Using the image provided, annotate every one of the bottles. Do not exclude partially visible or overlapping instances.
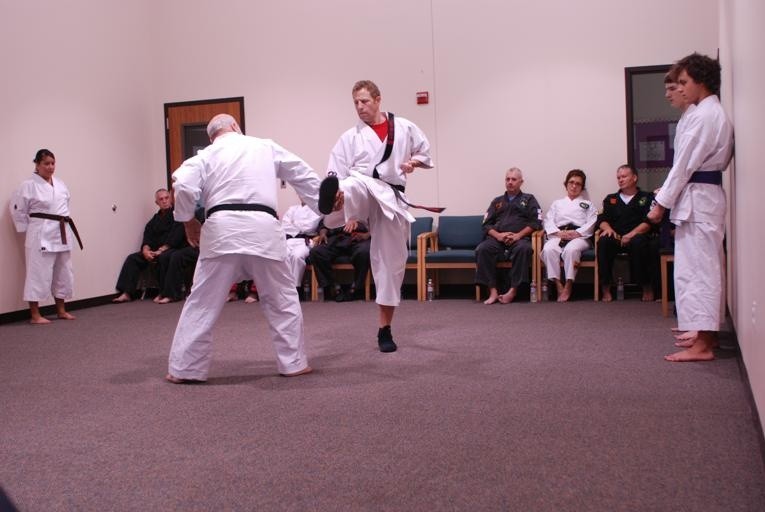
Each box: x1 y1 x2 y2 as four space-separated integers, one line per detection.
318 284 324 302
304 280 311 301
425 277 434 301
541 277 549 302
530 280 538 305
616 277 625 300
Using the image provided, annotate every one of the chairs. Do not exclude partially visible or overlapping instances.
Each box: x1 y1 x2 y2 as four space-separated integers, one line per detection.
312 255 366 303
478 215 537 304
365 217 433 302
537 226 602 303
610 252 644 296
660 246 675 307
422 213 486 302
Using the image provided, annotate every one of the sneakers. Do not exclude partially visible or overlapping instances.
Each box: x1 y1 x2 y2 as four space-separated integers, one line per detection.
378 325 397 352
318 171 338 214
348 280 359 301
331 284 345 302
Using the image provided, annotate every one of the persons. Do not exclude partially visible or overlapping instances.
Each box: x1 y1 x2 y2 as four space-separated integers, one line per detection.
474 168 543 305
282 192 322 300
226 279 260 304
9 147 83 325
157 206 206 304
112 187 174 304
166 113 343 385
597 163 658 304
537 168 600 302
309 198 373 303
664 53 737 364
315 79 433 354
662 70 699 341
